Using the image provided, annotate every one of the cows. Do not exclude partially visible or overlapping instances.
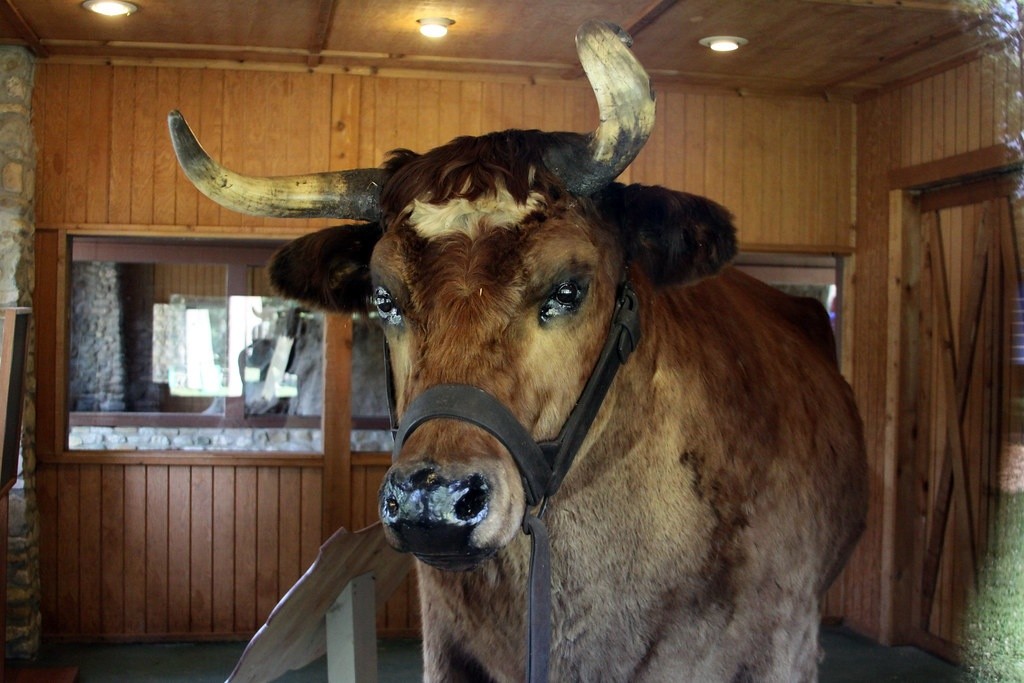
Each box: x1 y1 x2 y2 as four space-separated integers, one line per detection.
165 17 873 683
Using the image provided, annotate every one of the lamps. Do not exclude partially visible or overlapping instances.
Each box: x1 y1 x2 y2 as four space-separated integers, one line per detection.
698 36 749 52
415 16 456 38
